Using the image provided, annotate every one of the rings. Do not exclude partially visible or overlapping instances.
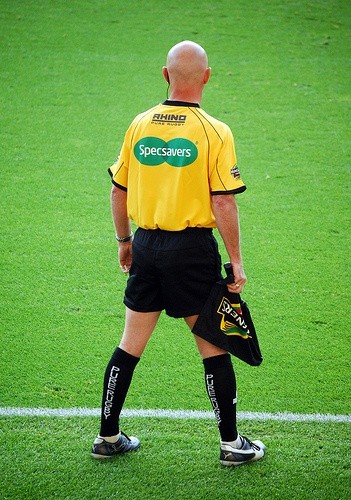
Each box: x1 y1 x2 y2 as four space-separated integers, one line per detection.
236 285 239 288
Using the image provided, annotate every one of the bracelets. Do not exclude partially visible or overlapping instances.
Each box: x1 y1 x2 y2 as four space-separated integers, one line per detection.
115 231 134 243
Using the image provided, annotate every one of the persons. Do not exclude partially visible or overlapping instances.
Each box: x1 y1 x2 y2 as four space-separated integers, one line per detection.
90 40 266 467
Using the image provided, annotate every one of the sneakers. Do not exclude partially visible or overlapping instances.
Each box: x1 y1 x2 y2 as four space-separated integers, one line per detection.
90 431 139 458
220 436 265 466
